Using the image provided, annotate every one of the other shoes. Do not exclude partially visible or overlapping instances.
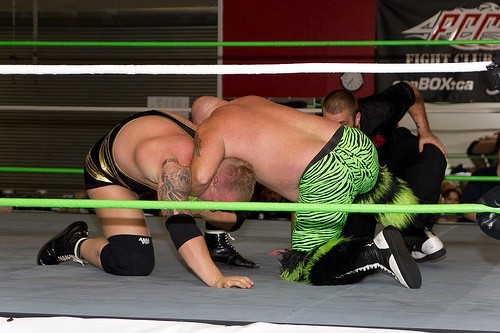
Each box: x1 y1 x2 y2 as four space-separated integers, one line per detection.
38 219 90 266
404 226 447 261
374 225 421 289
203 231 260 268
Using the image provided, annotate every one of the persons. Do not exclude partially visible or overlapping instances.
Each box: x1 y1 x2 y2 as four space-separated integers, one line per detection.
269 80 447 261
37 110 255 289
439 131 500 222
190 96 422 290
0 188 93 214
